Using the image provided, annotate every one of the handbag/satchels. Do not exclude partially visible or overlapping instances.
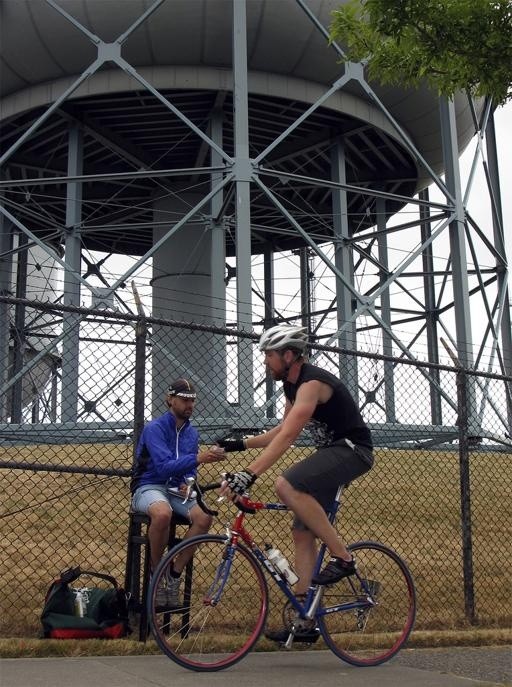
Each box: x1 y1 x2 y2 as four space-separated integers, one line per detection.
40 567 127 639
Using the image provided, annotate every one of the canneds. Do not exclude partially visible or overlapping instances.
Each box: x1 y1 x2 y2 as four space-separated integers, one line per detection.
74 597 87 618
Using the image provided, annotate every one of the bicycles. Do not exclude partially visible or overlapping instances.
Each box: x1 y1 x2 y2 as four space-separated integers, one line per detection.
145 478 418 673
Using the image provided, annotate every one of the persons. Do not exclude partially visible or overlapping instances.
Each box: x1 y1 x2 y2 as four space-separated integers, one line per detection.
129 378 225 607
213 324 375 643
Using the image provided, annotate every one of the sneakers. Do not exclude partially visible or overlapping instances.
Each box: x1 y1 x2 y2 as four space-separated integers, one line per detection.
166 564 183 609
310 555 357 585
150 567 167 612
264 624 320 644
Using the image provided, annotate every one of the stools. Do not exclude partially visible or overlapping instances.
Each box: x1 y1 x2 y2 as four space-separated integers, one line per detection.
126 512 194 642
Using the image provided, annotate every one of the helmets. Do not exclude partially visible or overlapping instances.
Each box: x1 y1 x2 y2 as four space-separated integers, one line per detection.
257 324 316 349
166 380 197 398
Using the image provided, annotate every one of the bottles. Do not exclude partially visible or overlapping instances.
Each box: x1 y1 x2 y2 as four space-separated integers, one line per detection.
263 543 301 588
